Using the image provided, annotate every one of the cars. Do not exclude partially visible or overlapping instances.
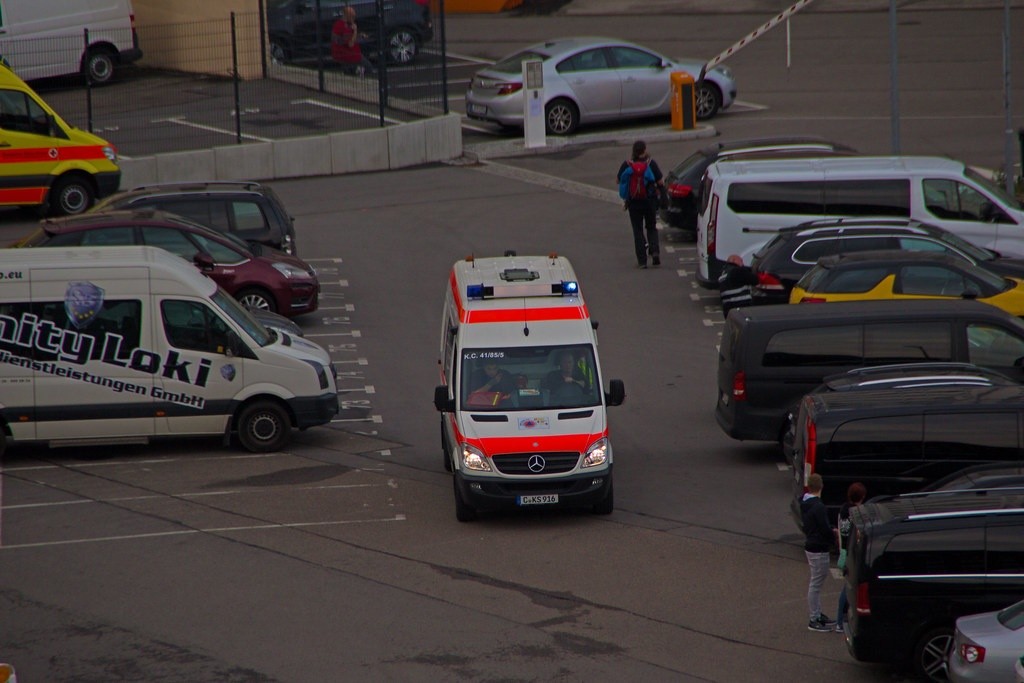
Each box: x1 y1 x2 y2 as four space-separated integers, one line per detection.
78 288 302 353
465 35 736 136
780 362 1024 683
659 136 886 228
790 250 1024 353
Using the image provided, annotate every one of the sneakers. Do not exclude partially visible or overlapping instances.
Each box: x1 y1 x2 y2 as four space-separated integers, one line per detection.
808 618 833 632
821 613 838 625
835 623 843 632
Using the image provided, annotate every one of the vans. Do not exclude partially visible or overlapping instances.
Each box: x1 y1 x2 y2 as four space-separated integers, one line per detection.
0 245 339 467
696 156 1024 291
714 301 1024 465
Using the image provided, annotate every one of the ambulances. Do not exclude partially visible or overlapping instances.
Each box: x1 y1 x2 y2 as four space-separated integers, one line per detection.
433 249 625 525
0 63 122 214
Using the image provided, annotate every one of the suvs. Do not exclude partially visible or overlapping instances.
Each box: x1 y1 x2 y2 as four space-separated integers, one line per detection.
752 216 1024 305
16 208 319 318
85 180 297 257
262 0 432 66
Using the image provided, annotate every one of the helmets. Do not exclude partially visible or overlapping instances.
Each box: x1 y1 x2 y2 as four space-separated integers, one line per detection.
343 7 355 16
727 255 743 267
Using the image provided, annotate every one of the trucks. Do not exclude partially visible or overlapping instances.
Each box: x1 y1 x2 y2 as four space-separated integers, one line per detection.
0 0 145 87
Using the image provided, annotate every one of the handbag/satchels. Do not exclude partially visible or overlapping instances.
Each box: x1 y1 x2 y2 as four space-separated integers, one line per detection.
465 391 503 407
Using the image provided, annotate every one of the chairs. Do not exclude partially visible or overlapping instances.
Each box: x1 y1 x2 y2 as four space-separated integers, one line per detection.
546 351 590 395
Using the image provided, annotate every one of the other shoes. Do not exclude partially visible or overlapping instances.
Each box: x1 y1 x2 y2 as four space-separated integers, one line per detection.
640 264 647 269
652 253 661 266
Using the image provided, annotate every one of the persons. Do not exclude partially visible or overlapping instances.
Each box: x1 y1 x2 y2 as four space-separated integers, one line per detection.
541 351 591 396
472 356 516 399
800 473 867 632
331 6 374 77
616 141 665 268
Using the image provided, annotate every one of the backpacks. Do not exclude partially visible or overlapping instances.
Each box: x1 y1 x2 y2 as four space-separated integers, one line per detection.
619 158 655 211
